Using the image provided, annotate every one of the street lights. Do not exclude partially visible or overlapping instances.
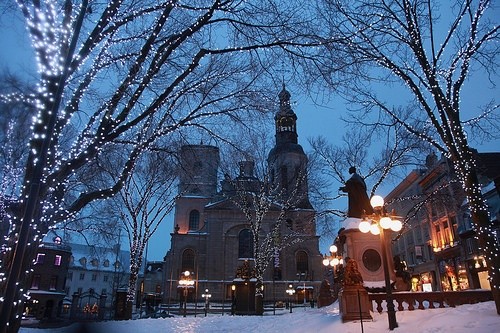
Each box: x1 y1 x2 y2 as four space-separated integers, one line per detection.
321 245 345 300
359 194 403 332
201 289 212 317
286 285 296 313
178 270 194 317
